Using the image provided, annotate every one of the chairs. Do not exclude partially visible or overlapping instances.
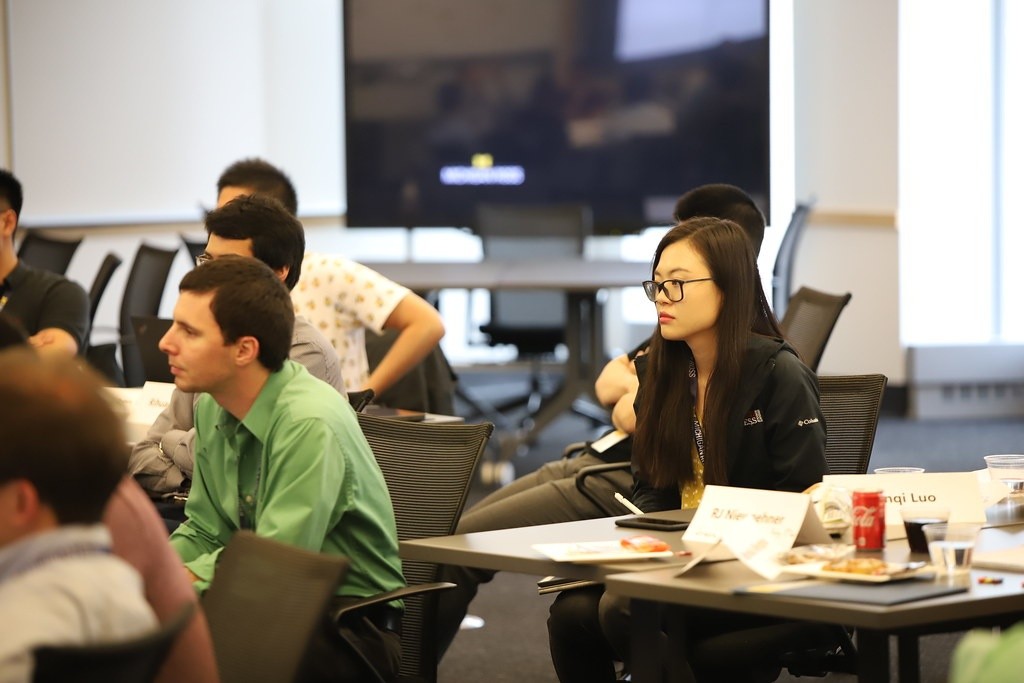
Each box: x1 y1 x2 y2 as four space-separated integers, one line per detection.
13 229 212 390
561 286 851 519
197 535 350 682
474 199 607 443
622 371 890 683
30 594 205 682
307 417 500 683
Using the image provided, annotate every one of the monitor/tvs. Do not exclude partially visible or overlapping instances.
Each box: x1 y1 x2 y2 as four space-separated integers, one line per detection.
342 0 771 230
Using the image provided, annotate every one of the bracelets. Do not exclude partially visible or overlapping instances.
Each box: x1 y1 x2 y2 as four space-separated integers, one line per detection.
159 440 164 456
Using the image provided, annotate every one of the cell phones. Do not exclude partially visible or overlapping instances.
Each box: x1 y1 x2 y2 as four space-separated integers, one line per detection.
616 517 691 531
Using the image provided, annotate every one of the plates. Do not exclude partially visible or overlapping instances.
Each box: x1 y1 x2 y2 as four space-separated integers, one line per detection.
779 558 927 582
534 538 673 565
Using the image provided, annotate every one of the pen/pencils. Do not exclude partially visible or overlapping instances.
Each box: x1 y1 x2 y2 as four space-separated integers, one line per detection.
615 492 644 514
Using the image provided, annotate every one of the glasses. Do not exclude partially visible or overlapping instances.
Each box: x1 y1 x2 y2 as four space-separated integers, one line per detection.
196 255 208 266
643 278 711 304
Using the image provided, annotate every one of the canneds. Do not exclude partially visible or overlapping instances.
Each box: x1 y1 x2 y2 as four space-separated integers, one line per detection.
852 488 886 551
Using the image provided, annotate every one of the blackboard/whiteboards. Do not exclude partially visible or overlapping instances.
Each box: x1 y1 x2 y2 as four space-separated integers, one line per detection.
0 0 352 236
793 0 897 228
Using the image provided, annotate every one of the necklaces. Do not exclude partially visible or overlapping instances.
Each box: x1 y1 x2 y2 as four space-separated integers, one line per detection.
238 442 261 529
689 353 706 467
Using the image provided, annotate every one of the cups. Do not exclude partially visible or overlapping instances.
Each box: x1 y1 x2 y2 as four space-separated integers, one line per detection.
874 466 950 552
923 522 980 585
984 453 1024 506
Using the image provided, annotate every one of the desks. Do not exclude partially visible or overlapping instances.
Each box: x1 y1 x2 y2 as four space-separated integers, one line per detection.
399 471 1024 683
372 257 657 459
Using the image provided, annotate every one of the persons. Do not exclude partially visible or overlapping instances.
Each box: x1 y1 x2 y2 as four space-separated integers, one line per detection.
0 169 218 683
424 184 765 665
129 158 445 536
159 256 407 683
547 217 827 683
